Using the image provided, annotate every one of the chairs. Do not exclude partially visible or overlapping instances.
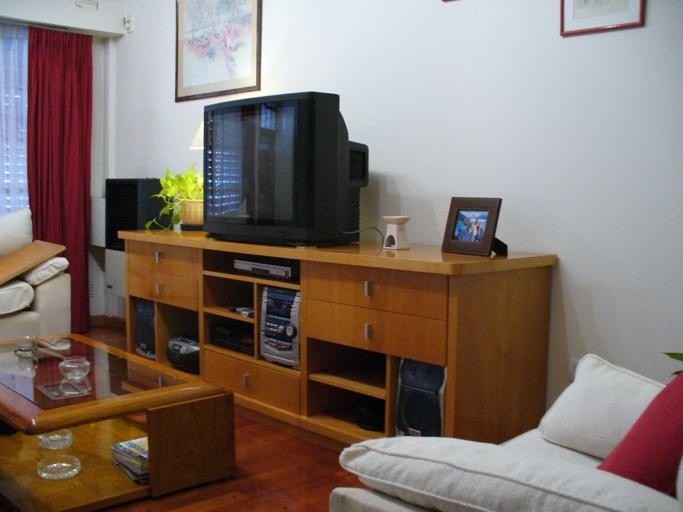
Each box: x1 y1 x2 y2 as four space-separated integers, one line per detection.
329 353 683 511
0 206 72 345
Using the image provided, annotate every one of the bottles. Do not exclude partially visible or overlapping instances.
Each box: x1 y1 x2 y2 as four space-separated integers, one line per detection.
59 379 94 398
58 356 92 381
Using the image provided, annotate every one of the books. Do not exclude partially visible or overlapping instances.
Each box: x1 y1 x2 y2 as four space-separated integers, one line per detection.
111 435 149 484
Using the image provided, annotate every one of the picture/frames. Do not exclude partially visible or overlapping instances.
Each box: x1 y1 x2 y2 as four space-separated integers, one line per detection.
559 0 646 37
440 197 508 258
175 0 262 103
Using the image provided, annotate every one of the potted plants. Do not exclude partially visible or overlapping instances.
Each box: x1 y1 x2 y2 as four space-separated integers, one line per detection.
145 162 204 229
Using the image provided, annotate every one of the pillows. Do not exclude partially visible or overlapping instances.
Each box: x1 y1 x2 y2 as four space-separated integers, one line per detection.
595 370 683 499
538 352 667 462
23 257 70 285
0 280 35 316
339 435 683 511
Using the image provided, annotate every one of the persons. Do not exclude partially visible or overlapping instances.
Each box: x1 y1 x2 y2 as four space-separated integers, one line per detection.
455 217 465 240
469 223 482 242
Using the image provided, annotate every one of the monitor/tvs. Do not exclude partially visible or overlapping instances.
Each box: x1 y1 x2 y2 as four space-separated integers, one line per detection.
202 90 369 249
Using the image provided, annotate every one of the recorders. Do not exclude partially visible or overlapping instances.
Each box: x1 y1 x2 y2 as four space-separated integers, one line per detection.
167 335 200 374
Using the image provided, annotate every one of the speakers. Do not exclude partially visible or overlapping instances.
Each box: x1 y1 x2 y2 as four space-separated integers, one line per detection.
394 358 446 437
134 298 156 360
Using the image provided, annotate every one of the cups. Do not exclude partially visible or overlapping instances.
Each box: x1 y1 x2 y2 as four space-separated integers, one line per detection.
17 334 39 352
17 354 40 373
38 429 75 468
